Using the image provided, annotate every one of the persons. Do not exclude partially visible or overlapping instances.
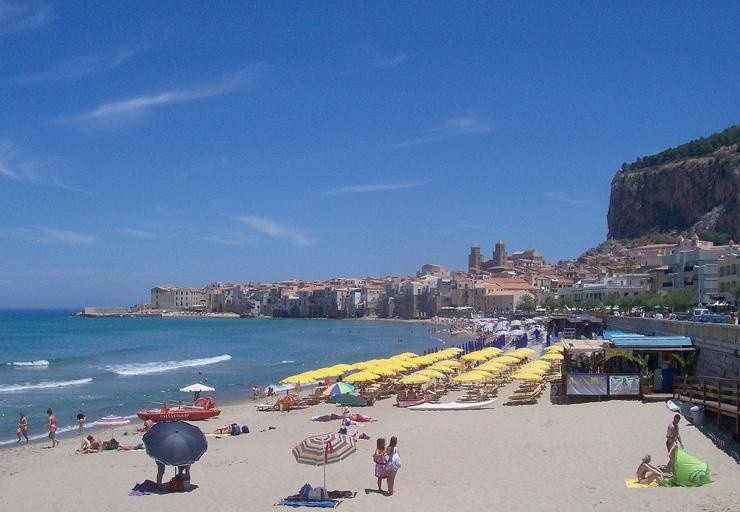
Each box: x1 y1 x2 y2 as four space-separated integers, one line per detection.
252 386 275 400
204 395 216 410
636 414 685 486
337 405 372 440
374 438 389 492
178 466 190 475
203 378 208 386
76 403 169 454
388 437 401 495
728 312 738 325
16 412 28 443
46 407 60 448
698 300 702 309
155 460 165 485
286 397 292 412
213 423 240 436
616 377 638 394
609 309 678 321
195 392 200 400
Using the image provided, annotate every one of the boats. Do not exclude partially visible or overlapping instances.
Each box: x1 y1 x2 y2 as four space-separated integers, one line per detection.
134 396 222 423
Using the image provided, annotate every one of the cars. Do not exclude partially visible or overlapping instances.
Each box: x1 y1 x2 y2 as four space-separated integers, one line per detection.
535 302 733 323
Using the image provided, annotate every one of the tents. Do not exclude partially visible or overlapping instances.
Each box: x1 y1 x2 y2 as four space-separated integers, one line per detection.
664 445 711 488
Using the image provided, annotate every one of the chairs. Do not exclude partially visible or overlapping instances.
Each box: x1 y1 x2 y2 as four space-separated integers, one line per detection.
254 369 548 413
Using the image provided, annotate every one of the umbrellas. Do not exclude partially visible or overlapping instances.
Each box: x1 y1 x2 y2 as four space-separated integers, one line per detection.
291 432 356 488
279 316 569 396
141 421 208 467
179 383 215 397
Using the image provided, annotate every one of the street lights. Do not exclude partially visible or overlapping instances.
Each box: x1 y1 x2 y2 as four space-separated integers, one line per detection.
692 264 706 308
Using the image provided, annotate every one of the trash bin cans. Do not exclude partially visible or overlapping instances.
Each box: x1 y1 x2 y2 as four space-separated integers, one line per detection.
691 405 706 426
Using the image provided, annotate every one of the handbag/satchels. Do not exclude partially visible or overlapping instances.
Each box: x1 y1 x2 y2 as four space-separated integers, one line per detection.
170 480 183 491
231 423 240 435
242 425 249 433
392 453 401 469
103 439 119 450
299 484 329 500
